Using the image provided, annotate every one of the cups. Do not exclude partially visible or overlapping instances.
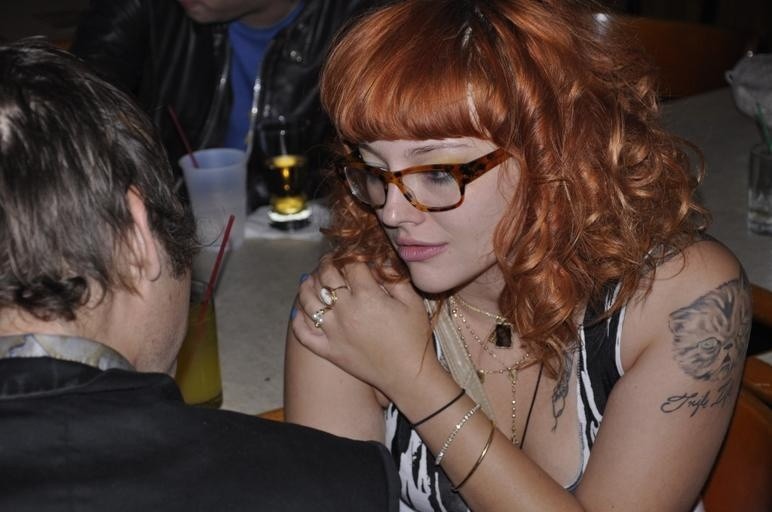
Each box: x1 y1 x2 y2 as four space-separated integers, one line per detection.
178 146 252 253
263 143 310 216
743 141 772 236
173 276 225 411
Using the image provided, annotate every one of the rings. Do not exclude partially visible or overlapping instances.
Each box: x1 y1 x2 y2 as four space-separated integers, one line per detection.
313 306 332 327
318 285 348 306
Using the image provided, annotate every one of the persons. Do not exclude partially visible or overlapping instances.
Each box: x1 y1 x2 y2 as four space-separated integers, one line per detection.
73 0 396 214
1 34 402 512
282 0 755 510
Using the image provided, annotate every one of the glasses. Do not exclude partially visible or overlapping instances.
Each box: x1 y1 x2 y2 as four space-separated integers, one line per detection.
337 146 511 212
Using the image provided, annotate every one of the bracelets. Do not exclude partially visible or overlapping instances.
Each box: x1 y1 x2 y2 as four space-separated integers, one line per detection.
435 404 482 466
409 389 466 430
451 421 496 492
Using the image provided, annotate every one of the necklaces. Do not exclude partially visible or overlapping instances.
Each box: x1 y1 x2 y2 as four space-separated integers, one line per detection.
450 295 549 451
